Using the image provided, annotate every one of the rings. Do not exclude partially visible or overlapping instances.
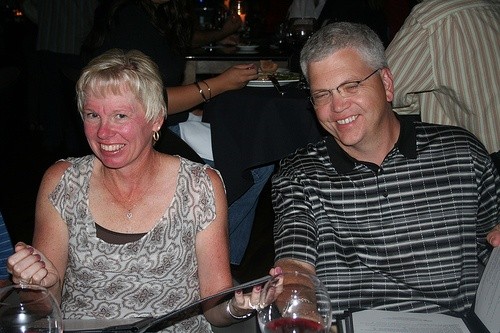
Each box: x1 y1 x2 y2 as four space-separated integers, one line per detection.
18 279 29 290
249 300 260 310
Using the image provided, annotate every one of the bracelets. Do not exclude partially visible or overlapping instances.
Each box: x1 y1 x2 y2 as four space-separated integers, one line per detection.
281 297 317 317
208 32 213 43
220 28 228 37
193 81 210 102
202 80 212 100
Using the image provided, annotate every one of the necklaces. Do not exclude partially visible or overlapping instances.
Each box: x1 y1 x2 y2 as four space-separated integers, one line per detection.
104 149 161 219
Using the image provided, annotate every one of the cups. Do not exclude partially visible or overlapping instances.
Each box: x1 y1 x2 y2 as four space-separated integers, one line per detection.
0 284 64 333
257 271 332 333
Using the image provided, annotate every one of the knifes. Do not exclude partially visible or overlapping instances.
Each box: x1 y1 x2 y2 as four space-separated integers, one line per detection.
267 75 286 95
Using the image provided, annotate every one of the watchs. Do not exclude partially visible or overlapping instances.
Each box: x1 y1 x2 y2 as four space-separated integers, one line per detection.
227 299 252 319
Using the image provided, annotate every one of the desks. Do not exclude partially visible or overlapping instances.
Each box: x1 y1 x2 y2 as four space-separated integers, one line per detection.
196 73 324 189
182 51 290 85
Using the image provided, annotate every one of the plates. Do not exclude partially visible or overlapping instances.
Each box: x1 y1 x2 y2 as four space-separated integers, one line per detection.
246 67 300 87
236 45 260 50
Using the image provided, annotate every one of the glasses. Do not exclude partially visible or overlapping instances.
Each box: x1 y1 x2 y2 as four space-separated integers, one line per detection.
308 68 381 106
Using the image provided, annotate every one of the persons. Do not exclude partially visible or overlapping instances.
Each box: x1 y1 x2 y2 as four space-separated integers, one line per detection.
0 0 500 333
